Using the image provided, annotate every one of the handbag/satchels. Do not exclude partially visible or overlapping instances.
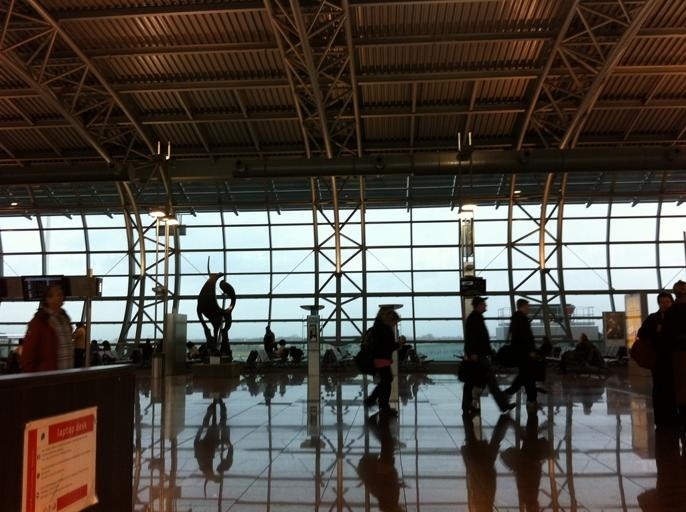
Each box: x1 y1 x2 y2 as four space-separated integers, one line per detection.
630 340 655 371
457 360 487 389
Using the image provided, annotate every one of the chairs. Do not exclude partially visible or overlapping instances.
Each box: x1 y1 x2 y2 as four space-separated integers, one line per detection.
459 344 633 386
235 346 429 388
96 349 196 388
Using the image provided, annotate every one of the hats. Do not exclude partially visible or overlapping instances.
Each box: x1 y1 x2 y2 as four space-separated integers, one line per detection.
472 297 487 304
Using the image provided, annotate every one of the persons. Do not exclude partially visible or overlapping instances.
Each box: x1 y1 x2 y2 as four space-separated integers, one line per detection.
661 280 686 351
458 411 511 511
373 413 404 511
136 370 293 403
20 284 73 370
132 341 199 363
520 403 544 511
637 426 686 512
7 322 116 366
461 296 517 413
631 291 674 425
246 373 303 406
247 327 304 368
543 373 607 414
363 308 398 414
399 374 434 405
539 334 594 370
399 335 411 360
500 298 543 407
